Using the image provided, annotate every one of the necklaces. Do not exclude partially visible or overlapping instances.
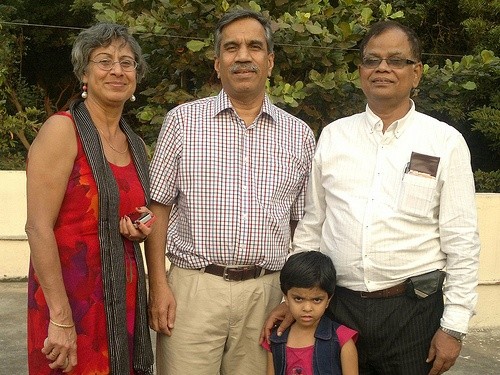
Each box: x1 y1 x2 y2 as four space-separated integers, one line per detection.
94 123 129 153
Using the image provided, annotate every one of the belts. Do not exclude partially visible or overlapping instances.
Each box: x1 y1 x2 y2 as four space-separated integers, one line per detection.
335 281 408 298
198 264 275 281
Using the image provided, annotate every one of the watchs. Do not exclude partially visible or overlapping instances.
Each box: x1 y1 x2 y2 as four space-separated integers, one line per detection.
439 325 466 342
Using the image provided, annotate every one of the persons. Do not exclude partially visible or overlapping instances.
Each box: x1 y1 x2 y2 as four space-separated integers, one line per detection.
25 23 152 375
259 20 482 375
261 251 360 375
145 9 316 375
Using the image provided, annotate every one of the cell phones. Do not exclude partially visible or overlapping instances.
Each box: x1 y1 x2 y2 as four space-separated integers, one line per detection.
128 212 152 228
145 216 157 227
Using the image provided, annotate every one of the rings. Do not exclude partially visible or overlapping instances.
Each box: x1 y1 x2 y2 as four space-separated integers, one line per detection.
129 235 134 240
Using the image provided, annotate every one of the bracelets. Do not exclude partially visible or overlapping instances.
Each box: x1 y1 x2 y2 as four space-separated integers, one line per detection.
49 320 75 328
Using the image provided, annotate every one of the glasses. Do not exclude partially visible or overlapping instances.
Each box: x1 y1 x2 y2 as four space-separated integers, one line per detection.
362 54 416 69
89 58 139 72
